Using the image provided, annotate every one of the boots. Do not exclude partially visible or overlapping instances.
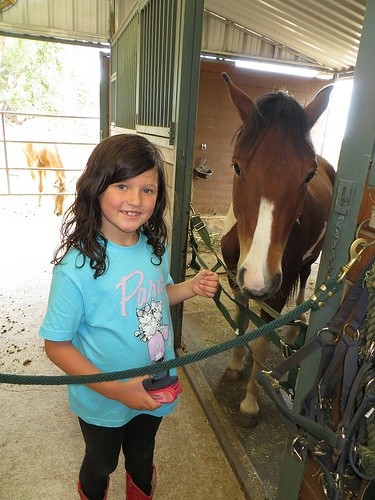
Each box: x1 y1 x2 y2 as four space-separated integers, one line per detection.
77 477 111 499
125 462 157 499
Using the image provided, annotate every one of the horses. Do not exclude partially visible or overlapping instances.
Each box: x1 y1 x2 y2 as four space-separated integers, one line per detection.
216 71 339 430
22 135 70 216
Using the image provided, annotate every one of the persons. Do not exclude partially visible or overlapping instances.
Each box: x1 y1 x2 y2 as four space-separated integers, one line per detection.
37 133 219 500
